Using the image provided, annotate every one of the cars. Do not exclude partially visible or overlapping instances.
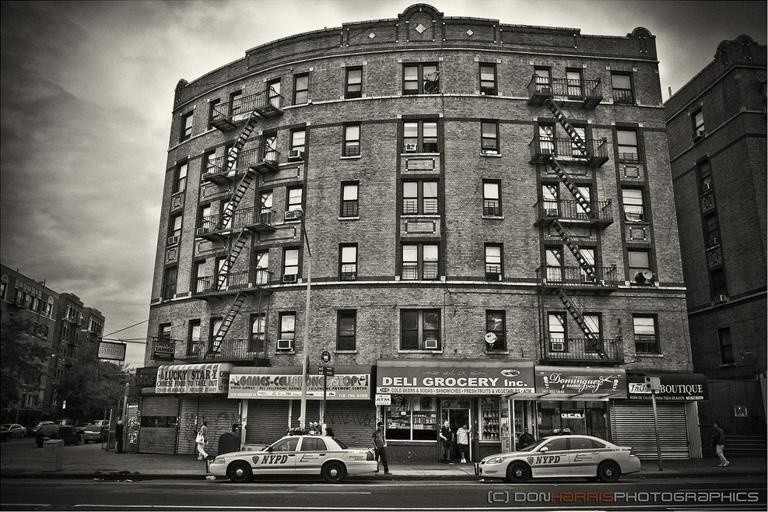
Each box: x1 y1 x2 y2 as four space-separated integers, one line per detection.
88 419 109 425
475 436 641 483
29 421 54 436
83 425 109 444
36 424 81 447
205 434 380 484
0 424 28 442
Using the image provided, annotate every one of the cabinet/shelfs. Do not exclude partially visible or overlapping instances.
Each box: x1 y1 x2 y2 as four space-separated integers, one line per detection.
477 396 502 444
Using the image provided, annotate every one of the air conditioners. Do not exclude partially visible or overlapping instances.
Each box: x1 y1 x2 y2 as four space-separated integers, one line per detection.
715 295 728 304
168 88 562 351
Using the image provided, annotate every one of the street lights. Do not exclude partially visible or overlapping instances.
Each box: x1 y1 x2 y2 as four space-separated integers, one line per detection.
293 208 312 432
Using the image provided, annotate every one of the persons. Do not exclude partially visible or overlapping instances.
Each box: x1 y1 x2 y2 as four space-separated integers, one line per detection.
519 427 534 448
439 420 455 463
326 427 334 436
713 423 730 467
231 423 240 450
456 423 472 464
197 422 208 461
372 422 392 475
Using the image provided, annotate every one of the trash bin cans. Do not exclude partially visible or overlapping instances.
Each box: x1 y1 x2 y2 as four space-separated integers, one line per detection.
218 432 241 457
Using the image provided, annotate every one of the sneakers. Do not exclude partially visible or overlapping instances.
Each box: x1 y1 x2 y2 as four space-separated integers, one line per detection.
718 461 730 467
461 458 466 464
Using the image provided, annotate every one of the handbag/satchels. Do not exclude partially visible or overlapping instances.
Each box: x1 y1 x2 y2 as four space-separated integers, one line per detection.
196 435 204 444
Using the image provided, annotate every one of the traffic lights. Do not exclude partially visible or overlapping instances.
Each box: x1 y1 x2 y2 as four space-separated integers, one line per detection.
318 367 334 377
645 377 661 390
